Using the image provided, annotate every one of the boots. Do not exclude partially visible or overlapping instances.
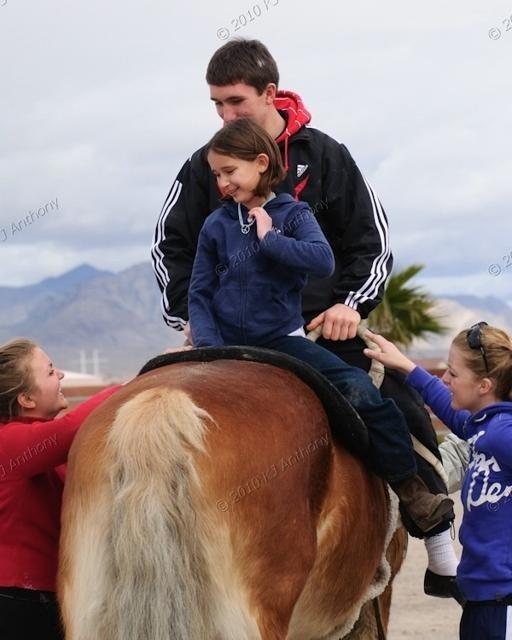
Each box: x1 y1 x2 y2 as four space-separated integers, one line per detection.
391 472 454 533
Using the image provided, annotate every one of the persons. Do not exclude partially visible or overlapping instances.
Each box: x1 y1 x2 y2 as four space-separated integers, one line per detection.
1 338 135 640
187 117 454 532
363 321 512 640
148 39 463 598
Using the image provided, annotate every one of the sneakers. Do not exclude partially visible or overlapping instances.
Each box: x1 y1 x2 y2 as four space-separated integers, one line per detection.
423 567 459 599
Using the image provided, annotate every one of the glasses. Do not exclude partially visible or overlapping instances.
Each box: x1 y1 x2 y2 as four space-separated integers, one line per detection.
466 322 489 378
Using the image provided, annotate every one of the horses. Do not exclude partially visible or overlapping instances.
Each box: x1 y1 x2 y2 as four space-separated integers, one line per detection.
55 356 408 640
